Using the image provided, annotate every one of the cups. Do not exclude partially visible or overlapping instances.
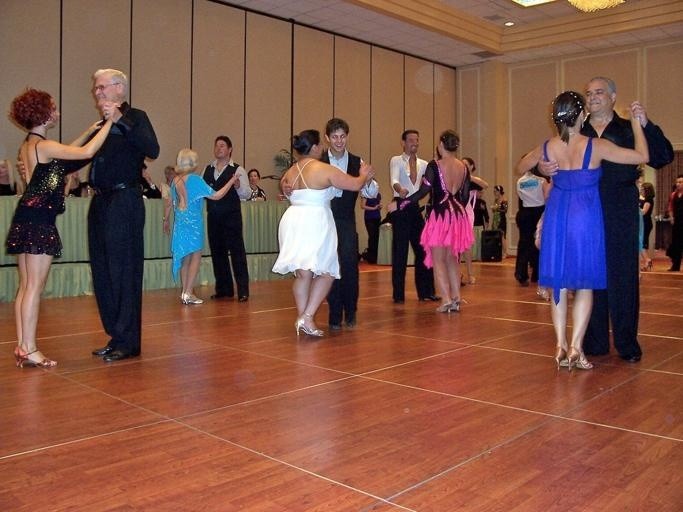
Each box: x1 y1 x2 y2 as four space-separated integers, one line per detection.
88 185 94 198
251 192 258 202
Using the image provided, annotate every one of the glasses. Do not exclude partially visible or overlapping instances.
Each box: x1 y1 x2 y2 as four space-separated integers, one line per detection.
93 82 118 92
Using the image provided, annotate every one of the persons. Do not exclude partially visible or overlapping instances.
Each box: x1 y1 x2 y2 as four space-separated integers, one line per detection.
271 130 373 339
538 76 674 361
15 69 160 362
1 158 266 201
517 91 650 374
280 117 374 332
3 87 120 369
515 153 554 284
634 173 682 272
360 178 382 265
199 137 252 302
162 149 241 304
387 129 508 314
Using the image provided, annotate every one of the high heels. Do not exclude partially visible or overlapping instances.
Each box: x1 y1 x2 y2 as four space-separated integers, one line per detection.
641 260 652 271
294 313 324 337
182 293 203 304
450 295 460 310
567 347 594 371
536 286 550 301
14 346 57 368
437 303 452 314
554 346 576 371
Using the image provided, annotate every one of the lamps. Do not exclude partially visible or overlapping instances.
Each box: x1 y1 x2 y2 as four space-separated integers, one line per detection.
568 0 625 14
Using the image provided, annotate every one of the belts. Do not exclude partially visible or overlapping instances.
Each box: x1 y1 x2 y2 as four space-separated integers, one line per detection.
93 181 126 194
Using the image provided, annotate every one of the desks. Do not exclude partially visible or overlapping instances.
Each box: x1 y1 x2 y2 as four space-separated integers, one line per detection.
378 223 484 265
1 196 291 303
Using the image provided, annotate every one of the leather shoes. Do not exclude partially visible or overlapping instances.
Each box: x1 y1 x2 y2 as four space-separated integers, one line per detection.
618 349 641 362
330 322 341 330
517 279 528 286
211 295 223 298
420 294 440 301
345 311 355 327
93 346 114 355
104 351 130 361
239 296 247 301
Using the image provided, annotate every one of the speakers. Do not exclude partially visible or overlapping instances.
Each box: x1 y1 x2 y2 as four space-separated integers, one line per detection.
482 230 502 262
